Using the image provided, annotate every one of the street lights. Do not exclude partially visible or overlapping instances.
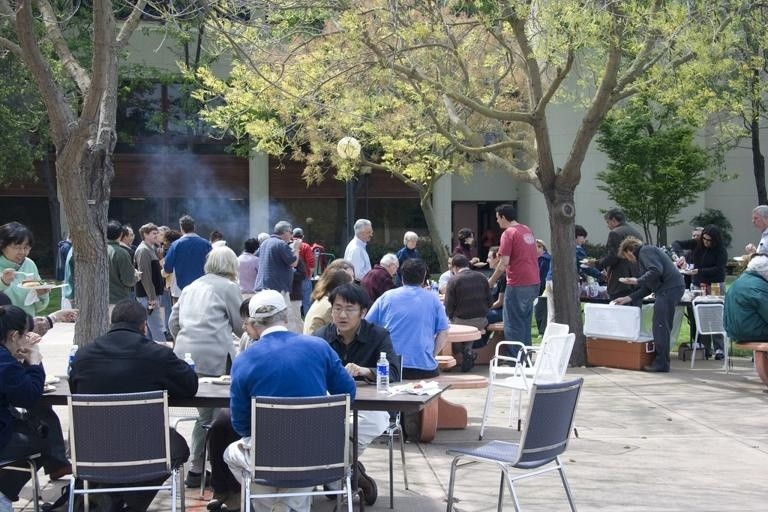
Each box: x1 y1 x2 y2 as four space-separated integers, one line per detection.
336 137 362 244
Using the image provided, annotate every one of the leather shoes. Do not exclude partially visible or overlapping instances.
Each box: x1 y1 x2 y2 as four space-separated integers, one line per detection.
461 347 477 373
351 461 377 506
643 364 669 372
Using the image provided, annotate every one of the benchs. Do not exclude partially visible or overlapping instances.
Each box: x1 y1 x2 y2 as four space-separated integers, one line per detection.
724 265 737 275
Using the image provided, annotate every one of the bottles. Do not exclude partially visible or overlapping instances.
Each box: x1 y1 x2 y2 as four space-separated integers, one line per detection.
432 282 439 297
66 345 78 380
183 352 195 371
377 352 389 393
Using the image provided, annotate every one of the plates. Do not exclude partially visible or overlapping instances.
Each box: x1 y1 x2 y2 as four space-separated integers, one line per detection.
474 263 487 266
579 259 590 268
733 257 745 261
17 283 68 289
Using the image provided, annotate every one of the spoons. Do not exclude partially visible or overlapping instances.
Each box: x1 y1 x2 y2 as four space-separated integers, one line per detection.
13 272 34 277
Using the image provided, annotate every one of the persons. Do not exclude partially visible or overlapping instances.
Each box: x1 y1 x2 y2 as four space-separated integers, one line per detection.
222 288 357 512
66 298 199 512
744 202 768 257
0 220 53 385
0 301 74 512
0 290 80 342
207 294 259 512
303 280 400 508
722 253 768 344
55 204 733 489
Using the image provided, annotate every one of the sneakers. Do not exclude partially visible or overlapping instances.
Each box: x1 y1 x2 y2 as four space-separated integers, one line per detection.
184 470 211 488
715 349 724 360
220 492 241 511
0 491 14 512
50 465 72 480
206 488 228 510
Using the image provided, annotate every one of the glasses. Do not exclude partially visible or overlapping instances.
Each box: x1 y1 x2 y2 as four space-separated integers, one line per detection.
702 236 712 241
7 245 32 251
19 332 31 342
332 304 361 313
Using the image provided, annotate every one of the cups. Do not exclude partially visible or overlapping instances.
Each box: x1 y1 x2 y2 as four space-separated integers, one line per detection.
710 283 721 298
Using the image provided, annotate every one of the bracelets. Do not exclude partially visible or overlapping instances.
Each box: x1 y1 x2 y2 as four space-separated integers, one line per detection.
50 313 58 323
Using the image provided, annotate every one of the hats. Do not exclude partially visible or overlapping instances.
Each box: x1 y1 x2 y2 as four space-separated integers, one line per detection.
292 227 303 237
248 288 286 318
